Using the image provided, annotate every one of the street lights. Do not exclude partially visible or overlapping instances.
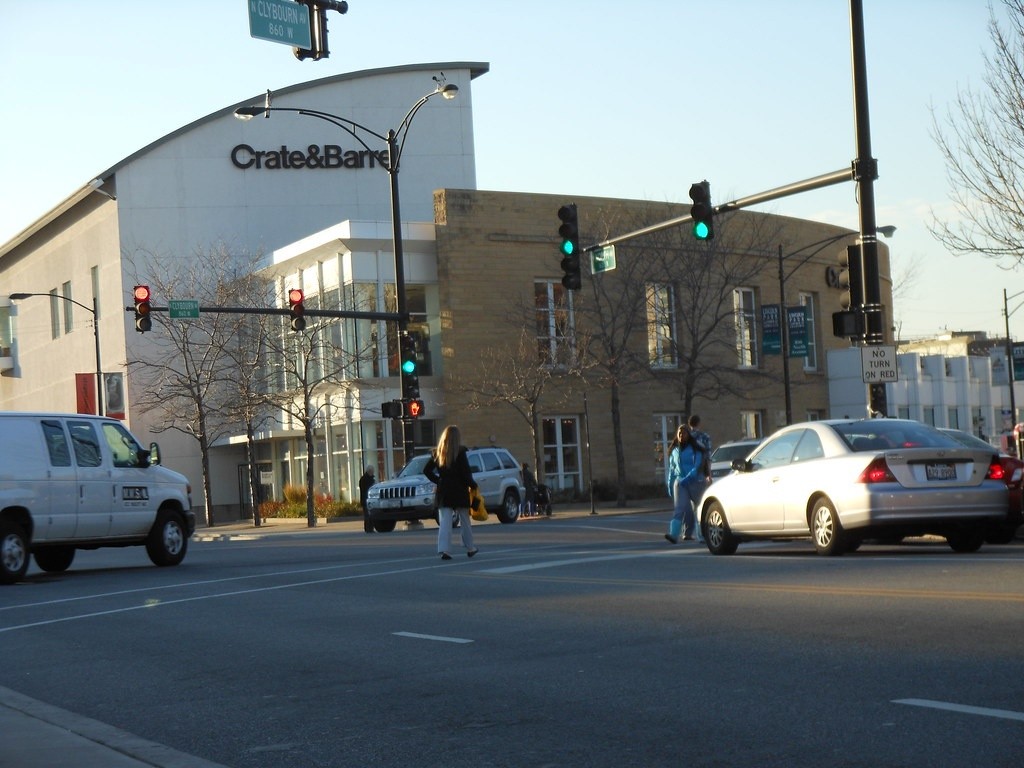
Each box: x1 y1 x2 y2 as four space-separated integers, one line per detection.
234 82 459 524
9 292 103 416
779 225 897 425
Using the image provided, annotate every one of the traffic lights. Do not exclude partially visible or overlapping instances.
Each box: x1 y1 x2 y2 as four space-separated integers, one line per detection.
408 400 425 417
558 203 581 290
134 286 152 332
832 310 865 336
839 245 864 310
400 335 420 399
289 289 306 331
689 179 715 241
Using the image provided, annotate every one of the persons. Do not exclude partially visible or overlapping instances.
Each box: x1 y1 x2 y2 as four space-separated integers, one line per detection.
663 414 711 544
359 465 376 535
424 426 478 560
520 463 537 518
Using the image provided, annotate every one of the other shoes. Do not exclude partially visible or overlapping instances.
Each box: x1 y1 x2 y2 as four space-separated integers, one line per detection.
442 553 457 559
468 546 480 557
683 535 692 539
665 534 679 543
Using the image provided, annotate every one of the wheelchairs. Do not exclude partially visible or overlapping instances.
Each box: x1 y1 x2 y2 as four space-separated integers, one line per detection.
523 484 553 516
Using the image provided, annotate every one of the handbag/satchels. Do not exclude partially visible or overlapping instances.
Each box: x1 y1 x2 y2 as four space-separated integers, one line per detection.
470 486 489 521
471 489 481 512
689 479 707 503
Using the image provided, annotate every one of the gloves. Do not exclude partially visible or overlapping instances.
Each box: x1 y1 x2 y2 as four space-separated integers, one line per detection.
680 477 690 487
668 487 673 496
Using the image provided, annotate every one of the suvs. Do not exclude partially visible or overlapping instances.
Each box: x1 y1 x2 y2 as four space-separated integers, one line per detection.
0 412 196 584
366 446 527 533
709 436 767 485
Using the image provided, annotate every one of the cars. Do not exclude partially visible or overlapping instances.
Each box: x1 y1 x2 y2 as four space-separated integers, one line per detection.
696 416 1010 558
862 427 1024 552
